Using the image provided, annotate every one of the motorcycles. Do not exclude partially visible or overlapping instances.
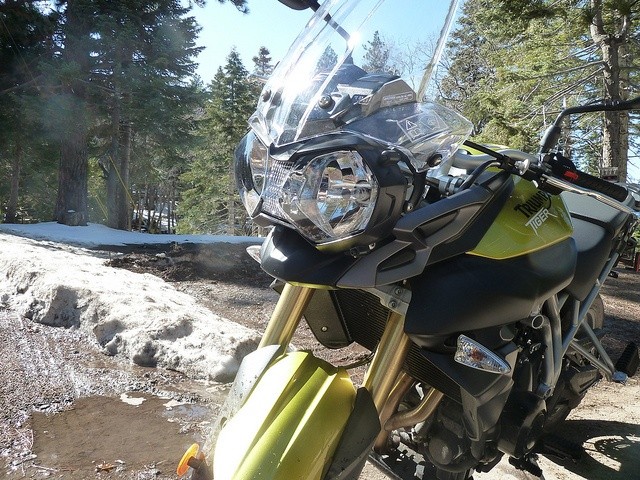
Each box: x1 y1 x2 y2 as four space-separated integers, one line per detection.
176 0 640 479
